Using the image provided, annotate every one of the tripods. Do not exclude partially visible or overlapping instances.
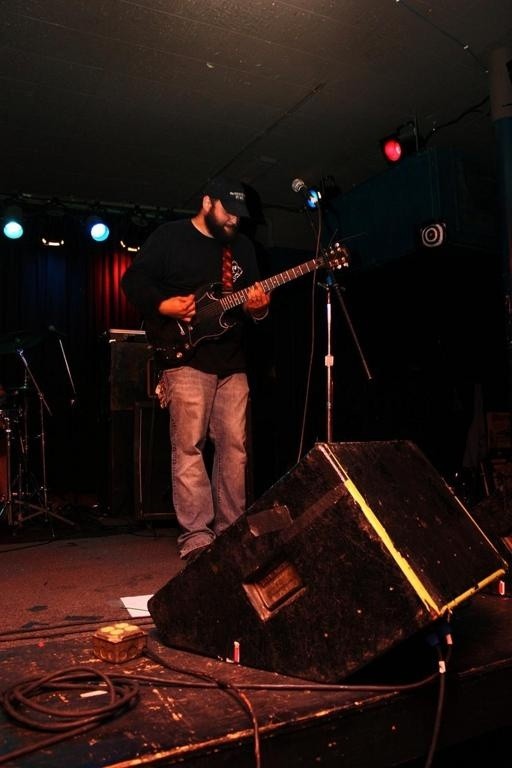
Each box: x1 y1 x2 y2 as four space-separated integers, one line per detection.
0 366 80 538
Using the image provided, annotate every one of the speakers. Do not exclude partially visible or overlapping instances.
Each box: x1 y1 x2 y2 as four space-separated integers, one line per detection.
109 341 160 411
146 438 512 686
470 487 512 597
109 406 176 520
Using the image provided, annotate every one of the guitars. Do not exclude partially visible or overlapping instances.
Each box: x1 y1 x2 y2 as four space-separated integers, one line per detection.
144 243 351 365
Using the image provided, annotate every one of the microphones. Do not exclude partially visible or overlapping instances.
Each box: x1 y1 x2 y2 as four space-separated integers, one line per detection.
291 178 320 209
48 324 69 337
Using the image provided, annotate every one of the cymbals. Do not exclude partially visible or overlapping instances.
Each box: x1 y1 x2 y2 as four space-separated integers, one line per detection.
6 386 39 399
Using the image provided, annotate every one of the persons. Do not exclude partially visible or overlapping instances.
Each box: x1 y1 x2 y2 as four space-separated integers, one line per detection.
118 175 276 559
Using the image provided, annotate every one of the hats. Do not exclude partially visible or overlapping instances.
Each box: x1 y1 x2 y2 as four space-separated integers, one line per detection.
209 177 249 217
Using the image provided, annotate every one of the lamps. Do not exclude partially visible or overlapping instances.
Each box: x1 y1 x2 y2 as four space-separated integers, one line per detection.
378 130 424 164
84 215 111 243
288 175 322 208
0 206 25 240
